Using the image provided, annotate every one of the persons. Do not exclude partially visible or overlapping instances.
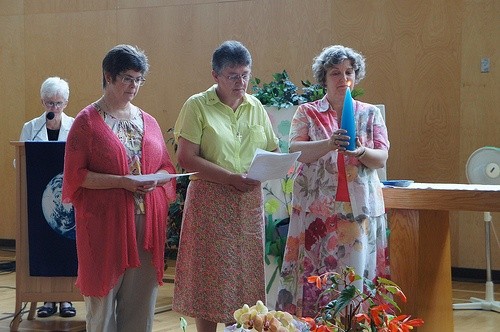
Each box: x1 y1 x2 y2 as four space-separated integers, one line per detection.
172 40 281 332
13 76 75 317
274 45 391 318
61 44 176 332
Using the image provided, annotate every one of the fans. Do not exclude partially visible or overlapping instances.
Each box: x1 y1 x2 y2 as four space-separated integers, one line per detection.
452 146 500 313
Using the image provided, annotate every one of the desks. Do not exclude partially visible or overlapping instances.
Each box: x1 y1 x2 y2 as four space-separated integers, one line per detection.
382 182 500 332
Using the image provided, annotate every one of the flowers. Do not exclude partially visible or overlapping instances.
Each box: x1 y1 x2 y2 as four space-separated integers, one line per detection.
178 265 424 332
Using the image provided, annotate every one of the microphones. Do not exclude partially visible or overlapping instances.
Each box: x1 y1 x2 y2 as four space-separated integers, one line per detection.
32 111 55 142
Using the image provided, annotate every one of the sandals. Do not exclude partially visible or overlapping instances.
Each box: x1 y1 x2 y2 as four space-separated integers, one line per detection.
60 301 76 317
37 301 57 317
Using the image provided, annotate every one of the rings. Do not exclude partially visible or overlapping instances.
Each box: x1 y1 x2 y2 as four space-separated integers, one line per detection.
334 139 338 145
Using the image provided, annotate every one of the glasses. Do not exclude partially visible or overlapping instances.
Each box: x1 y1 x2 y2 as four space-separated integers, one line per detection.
120 75 146 87
48 102 64 107
223 74 250 82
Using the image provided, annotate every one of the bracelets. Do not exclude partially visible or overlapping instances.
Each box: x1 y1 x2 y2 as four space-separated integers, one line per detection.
356 148 366 157
